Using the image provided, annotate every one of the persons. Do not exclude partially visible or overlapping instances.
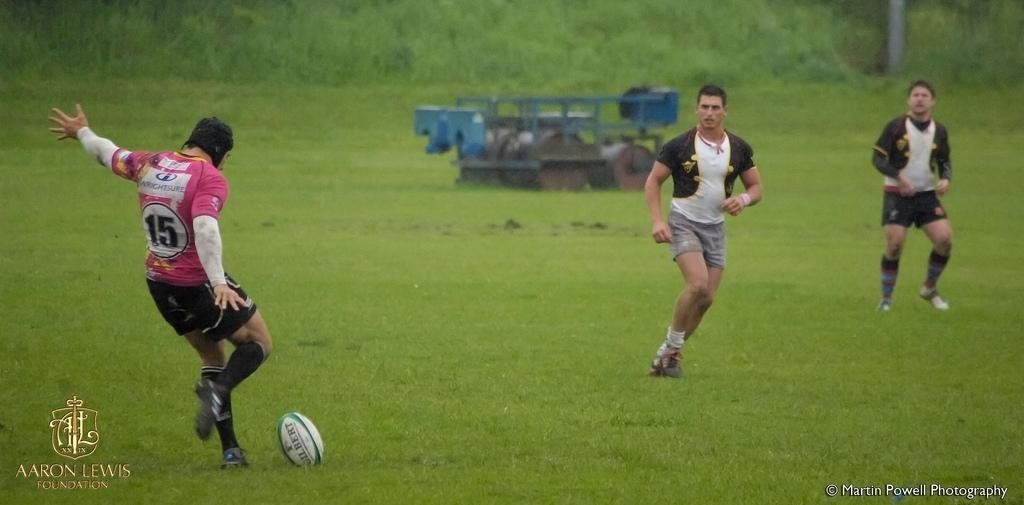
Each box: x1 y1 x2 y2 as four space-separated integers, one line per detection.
643 83 763 377
46 103 273 470
870 78 953 313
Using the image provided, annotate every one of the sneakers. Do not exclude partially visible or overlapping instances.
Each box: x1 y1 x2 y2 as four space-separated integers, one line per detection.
221 445 249 471
917 288 949 310
650 343 684 378
877 300 891 311
194 378 230 440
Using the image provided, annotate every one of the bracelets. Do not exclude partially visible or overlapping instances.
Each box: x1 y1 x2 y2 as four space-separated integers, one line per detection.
739 193 751 206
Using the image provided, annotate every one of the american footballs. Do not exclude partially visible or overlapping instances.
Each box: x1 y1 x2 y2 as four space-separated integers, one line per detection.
276 411 325 468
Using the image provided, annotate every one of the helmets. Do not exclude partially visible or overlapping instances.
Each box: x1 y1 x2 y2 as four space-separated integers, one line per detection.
186 125 234 164
